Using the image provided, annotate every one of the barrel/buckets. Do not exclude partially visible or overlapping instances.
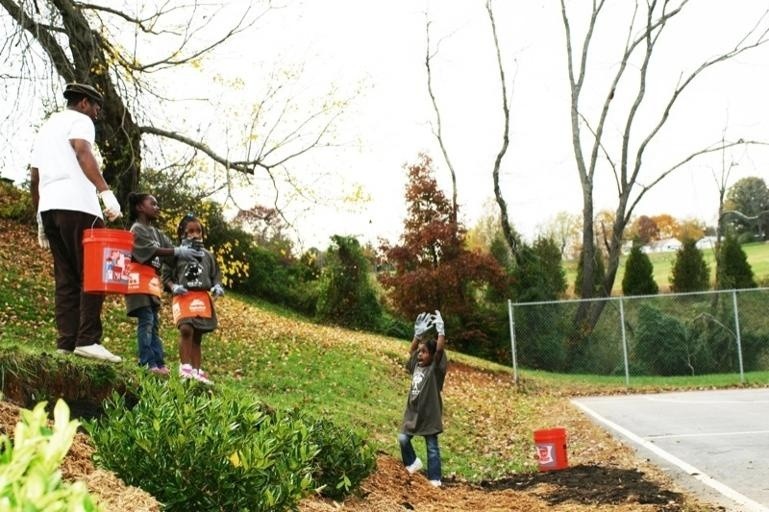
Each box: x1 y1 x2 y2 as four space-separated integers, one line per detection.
81 210 136 295
532 427 569 472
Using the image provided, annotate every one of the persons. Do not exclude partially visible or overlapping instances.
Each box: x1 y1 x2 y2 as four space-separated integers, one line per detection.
30 83 124 363
397 309 447 487
161 213 225 386
124 192 201 376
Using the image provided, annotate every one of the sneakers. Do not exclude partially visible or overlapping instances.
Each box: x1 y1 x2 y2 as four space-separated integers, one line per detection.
149 366 169 374
193 369 209 382
405 457 423 474
180 363 193 379
74 342 122 363
430 479 442 486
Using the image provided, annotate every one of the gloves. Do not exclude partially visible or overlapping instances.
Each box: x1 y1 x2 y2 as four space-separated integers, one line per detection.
99 190 123 223
210 283 224 301
38 220 51 250
171 283 188 296
431 309 445 336
414 311 433 341
174 243 205 264
